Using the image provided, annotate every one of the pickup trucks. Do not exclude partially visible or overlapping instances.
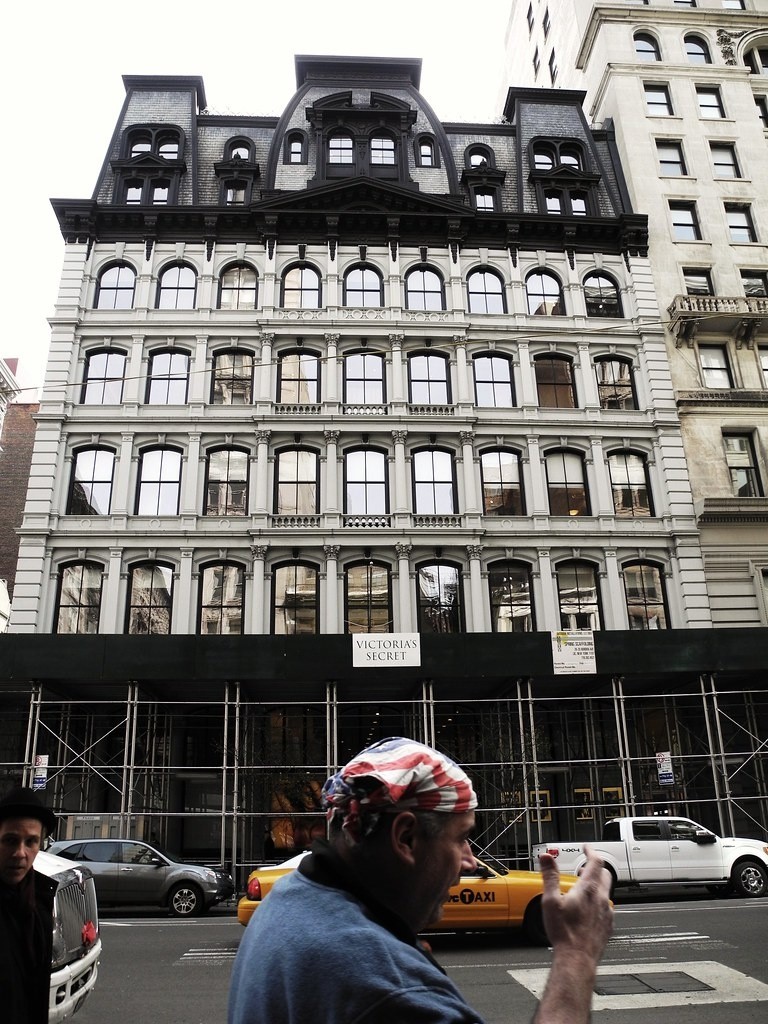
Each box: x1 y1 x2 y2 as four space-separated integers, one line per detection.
532 816 768 898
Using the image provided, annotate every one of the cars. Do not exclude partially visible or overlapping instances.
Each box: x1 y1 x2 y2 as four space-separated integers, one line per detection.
30 852 102 1024
237 849 615 952
46 838 235 918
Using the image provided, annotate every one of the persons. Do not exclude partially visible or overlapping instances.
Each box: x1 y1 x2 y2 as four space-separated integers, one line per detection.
227 736 614 1024
0 787 59 1024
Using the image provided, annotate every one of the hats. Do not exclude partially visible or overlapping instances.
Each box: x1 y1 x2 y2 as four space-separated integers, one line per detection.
0 789 57 840
